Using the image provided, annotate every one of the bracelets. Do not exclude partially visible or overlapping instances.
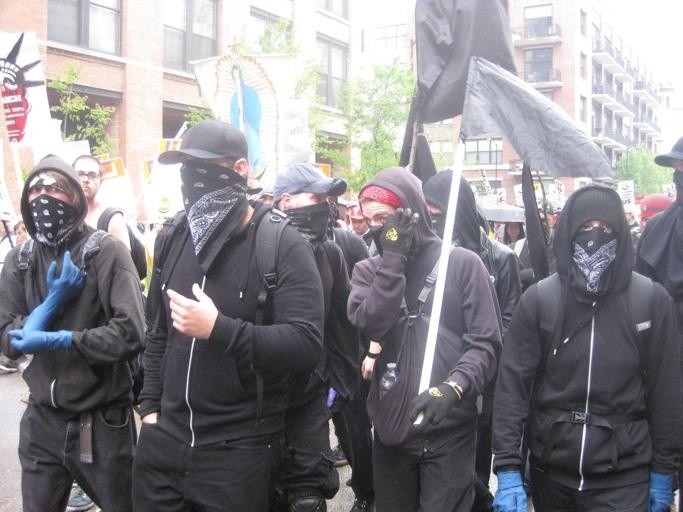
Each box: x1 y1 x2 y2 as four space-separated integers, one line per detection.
364 350 383 359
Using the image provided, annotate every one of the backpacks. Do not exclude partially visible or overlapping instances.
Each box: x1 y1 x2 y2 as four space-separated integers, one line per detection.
365 245 464 447
96 206 147 281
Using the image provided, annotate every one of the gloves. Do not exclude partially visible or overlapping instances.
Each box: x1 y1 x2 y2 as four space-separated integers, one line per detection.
491 471 528 512
649 470 674 512
19 251 85 331
379 208 419 258
410 380 463 432
7 329 72 354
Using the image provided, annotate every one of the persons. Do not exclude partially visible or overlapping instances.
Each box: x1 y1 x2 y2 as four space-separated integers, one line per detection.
491 183 680 511
474 194 680 285
226 63 263 172
272 160 380 511
346 163 525 511
0 152 148 511
635 135 680 302
0 211 29 373
130 118 324 508
64 153 132 268
255 189 277 207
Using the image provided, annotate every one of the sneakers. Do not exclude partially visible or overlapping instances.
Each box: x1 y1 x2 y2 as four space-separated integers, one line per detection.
66 489 95 512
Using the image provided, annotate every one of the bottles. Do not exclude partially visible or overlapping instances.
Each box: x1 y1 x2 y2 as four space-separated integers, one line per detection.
377 362 401 398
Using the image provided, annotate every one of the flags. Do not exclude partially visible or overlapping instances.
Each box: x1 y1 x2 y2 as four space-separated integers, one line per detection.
460 53 613 179
412 0 519 128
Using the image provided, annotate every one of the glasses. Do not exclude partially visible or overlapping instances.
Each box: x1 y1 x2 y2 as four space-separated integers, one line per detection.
77 172 98 181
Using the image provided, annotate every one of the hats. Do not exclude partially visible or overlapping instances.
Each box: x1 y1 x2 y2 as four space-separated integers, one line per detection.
526 199 552 213
28 171 82 212
273 163 401 219
655 137 683 167
639 194 672 218
568 189 622 240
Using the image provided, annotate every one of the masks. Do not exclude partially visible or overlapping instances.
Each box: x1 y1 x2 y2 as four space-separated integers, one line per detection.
158 119 248 164
